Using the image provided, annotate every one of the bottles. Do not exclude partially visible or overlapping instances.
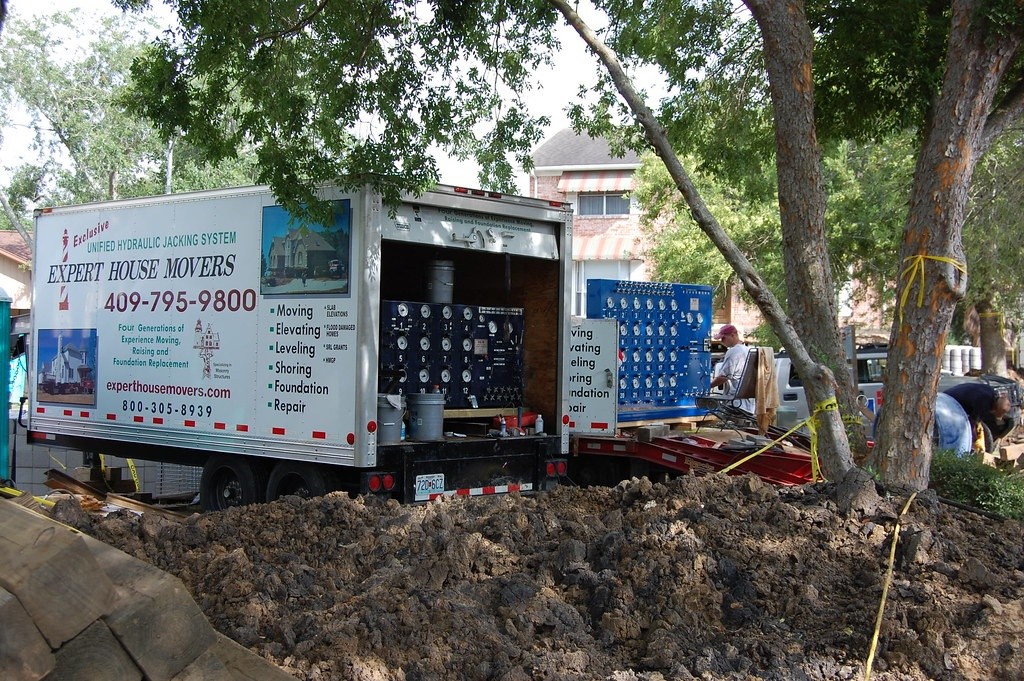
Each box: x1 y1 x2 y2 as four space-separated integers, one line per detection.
501 419 506 432
535 414 544 433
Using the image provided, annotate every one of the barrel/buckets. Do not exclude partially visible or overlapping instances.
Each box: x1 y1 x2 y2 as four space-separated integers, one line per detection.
407 392 446 441
375 393 406 442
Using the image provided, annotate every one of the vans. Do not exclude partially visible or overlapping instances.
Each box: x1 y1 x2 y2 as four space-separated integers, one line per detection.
712 342 1023 460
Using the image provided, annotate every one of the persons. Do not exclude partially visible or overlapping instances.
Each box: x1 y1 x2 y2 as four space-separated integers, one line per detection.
941 382 1011 455
710 325 756 427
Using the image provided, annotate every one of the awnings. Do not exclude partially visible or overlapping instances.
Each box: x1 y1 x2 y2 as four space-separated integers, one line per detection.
557 171 637 191
571 237 644 261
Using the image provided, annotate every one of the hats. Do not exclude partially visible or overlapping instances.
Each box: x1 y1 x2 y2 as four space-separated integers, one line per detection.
996 397 1011 421
714 325 737 339
